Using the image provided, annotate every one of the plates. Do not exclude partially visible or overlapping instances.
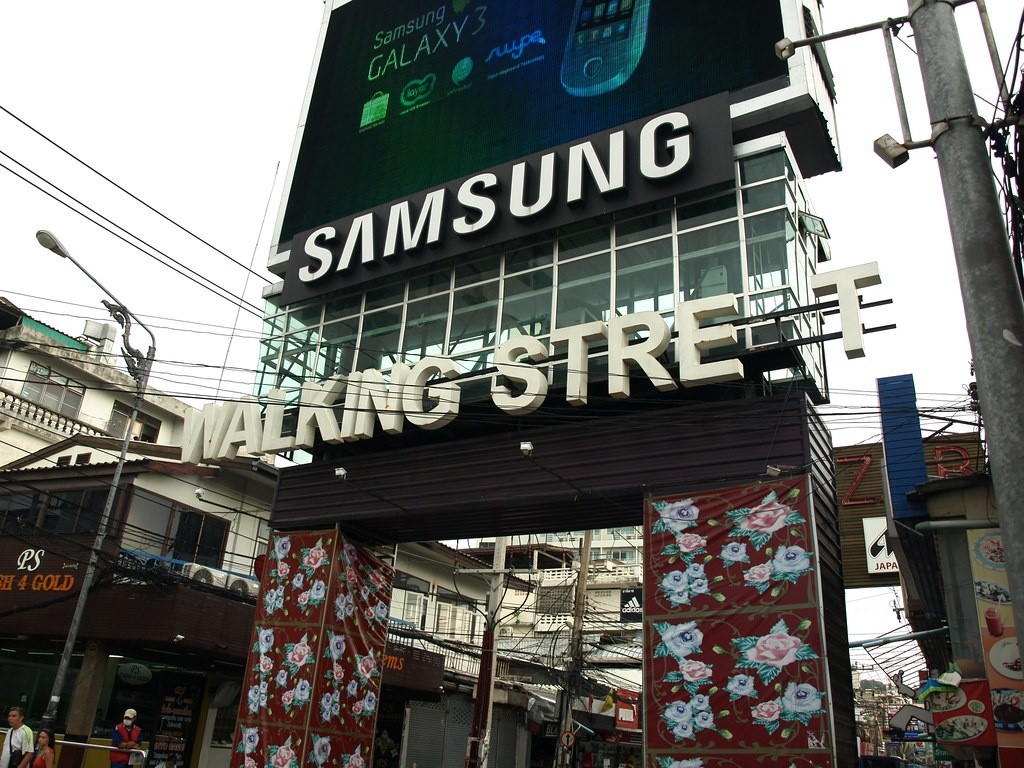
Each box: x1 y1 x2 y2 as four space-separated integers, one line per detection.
973 576 1011 605
934 715 989 743
972 530 1006 571
931 688 967 713
989 636 1023 680
990 687 1024 733
968 700 985 713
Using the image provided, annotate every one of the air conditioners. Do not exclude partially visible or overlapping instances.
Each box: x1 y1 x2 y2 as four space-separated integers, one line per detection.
226 574 261 600
181 563 228 589
500 626 513 640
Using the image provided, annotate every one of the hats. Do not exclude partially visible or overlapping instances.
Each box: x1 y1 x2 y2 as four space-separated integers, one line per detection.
124 709 137 718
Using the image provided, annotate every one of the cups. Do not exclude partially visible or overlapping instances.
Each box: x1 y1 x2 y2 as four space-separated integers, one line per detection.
983 610 1002 635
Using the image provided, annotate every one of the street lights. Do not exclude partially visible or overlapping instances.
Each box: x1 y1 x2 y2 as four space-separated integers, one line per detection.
28 229 157 768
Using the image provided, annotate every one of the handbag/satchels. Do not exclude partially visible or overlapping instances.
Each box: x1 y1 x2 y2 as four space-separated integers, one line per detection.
8 750 25 768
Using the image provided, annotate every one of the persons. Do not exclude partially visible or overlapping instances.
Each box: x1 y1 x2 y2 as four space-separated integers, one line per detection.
109 708 142 768
154 754 177 768
0 707 35 768
33 729 56 768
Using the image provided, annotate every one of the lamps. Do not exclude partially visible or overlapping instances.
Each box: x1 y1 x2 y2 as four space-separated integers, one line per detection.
334 468 408 510
519 441 584 494
766 464 781 477
195 488 269 522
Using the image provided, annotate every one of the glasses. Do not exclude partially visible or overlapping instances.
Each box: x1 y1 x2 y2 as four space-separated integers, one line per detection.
42 727 50 733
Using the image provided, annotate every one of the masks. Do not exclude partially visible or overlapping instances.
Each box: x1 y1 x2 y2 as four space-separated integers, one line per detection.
123 719 133 726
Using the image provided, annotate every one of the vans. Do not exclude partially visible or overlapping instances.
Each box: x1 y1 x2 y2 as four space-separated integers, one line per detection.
860 754 908 768
